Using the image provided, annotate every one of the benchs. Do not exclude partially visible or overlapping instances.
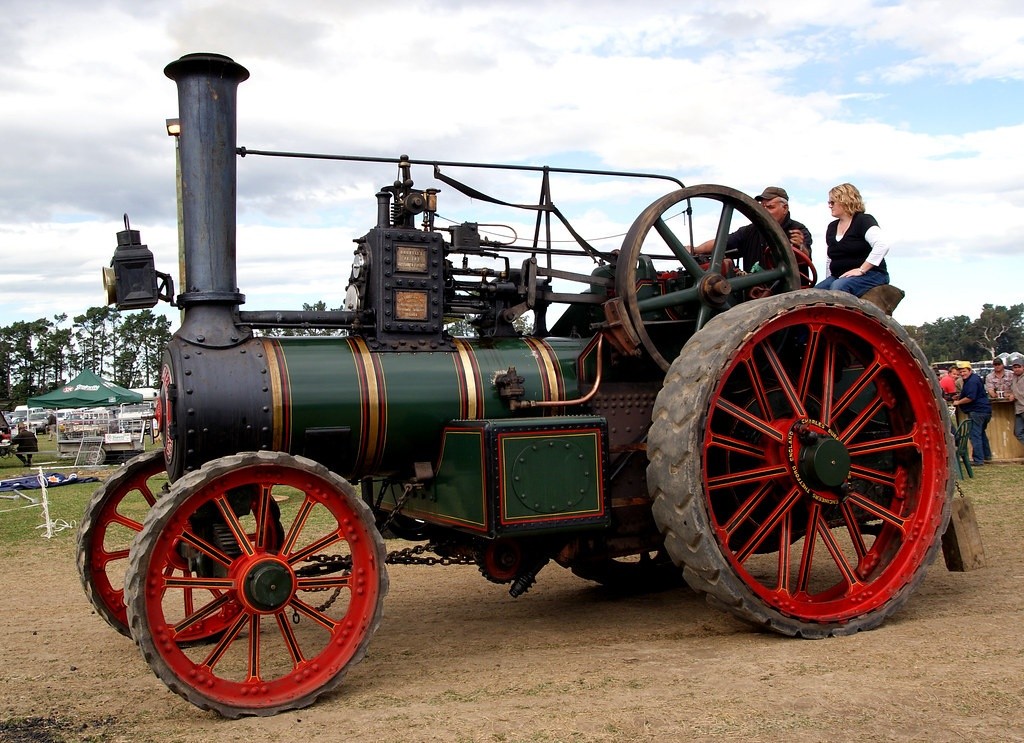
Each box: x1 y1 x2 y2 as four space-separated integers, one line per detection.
13 450 56 466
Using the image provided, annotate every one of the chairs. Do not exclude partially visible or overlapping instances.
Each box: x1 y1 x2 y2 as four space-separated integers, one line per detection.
952 418 974 478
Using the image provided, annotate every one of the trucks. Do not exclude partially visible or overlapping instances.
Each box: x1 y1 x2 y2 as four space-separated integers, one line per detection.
119 388 160 432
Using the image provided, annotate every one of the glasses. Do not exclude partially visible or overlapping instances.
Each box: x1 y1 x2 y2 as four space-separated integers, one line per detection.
828 201 840 205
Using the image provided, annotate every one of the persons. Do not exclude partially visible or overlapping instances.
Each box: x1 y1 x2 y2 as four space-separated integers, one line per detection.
810 182 891 298
10 420 39 468
681 186 816 298
938 357 1024 466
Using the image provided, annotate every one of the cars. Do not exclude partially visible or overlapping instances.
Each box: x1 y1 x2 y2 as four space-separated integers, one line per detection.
4 407 118 435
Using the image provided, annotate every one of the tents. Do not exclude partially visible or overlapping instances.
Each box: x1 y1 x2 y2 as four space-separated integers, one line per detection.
26 368 143 451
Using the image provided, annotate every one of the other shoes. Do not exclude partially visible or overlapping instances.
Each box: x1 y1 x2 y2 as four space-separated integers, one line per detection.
984 458 992 463
970 461 984 466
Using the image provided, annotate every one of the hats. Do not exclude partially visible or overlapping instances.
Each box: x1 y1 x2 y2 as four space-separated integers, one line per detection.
957 362 972 370
754 186 788 200
1012 359 1024 366
992 358 1003 365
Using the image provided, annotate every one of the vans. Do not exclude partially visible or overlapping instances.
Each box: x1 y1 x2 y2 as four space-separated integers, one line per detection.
11 405 43 423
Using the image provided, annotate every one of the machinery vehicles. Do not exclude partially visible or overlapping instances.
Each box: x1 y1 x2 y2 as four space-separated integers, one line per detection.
76 53 986 720
